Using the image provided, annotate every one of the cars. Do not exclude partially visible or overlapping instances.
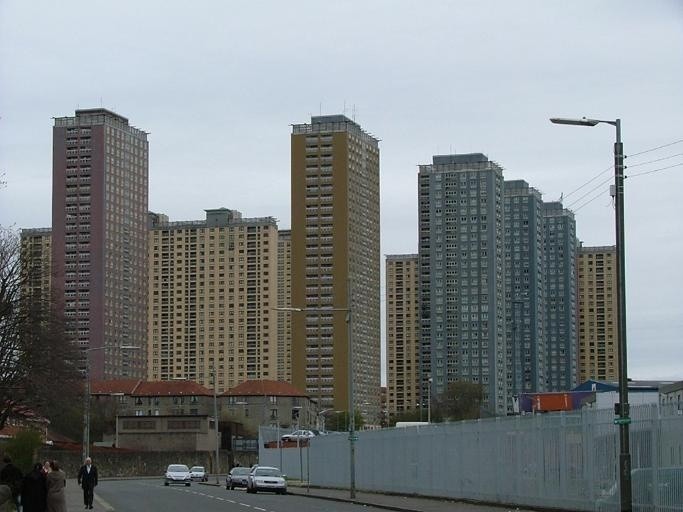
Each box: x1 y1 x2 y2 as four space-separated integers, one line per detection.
165 465 191 486
189 466 208 482
590 465 683 511
224 467 251 490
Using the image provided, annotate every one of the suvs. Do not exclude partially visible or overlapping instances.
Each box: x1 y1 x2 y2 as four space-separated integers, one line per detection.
247 464 286 496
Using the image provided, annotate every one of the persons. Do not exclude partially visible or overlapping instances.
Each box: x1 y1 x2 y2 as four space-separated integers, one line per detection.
0 455 66 512
78 457 98 509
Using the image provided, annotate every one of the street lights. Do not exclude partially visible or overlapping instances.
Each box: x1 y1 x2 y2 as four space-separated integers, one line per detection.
214 401 250 486
81 346 141 462
276 404 388 449
269 273 355 500
548 113 636 512
427 377 432 423
416 401 424 421
